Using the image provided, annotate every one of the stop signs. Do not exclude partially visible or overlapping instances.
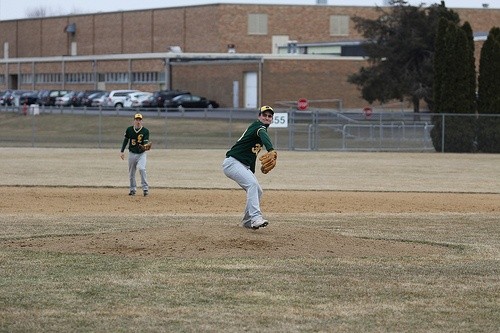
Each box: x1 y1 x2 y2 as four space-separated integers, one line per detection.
364 108 373 119
297 98 309 111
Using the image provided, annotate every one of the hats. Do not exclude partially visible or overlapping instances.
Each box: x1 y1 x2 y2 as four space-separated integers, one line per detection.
134 114 142 119
259 106 274 116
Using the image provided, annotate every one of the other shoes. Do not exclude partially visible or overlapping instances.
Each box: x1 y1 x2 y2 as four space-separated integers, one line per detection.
245 222 251 227
144 191 148 195
129 191 135 195
251 219 268 229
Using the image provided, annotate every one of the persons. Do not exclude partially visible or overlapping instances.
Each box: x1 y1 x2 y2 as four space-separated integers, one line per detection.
121 113 149 197
222 106 278 230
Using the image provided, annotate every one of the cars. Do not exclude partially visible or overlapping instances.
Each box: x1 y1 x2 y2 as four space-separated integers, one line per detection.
163 94 219 113
0 88 191 111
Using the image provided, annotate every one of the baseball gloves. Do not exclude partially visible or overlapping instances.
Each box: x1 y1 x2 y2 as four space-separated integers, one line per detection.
259 150 278 174
144 141 152 151
